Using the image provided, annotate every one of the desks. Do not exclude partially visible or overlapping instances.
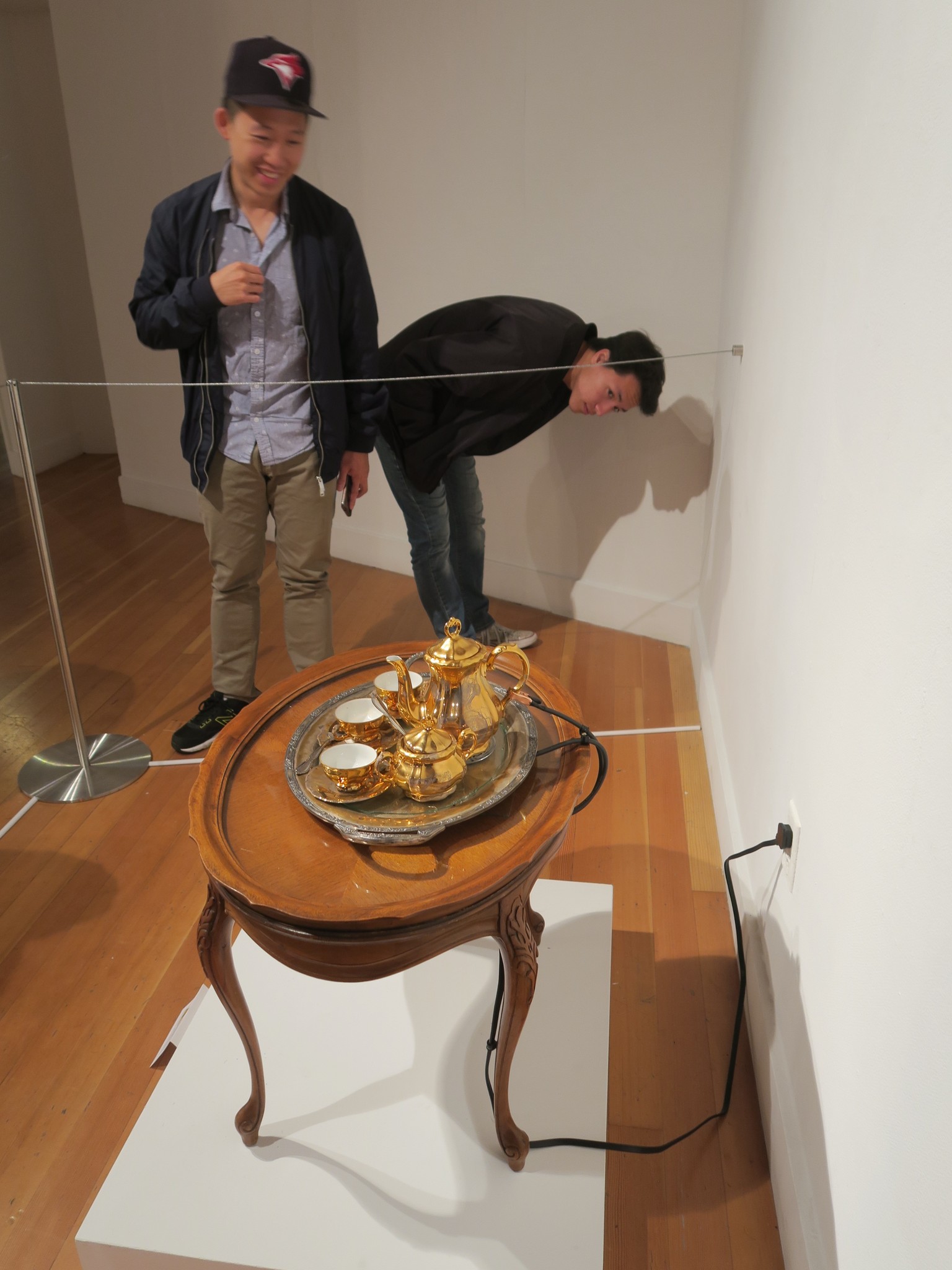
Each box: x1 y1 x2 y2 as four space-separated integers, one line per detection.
185 639 591 1176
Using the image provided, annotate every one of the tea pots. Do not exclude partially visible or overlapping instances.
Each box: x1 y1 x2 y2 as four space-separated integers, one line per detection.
386 618 530 756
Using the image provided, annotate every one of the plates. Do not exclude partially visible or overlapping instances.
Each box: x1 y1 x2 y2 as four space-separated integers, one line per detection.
361 719 401 750
305 763 391 803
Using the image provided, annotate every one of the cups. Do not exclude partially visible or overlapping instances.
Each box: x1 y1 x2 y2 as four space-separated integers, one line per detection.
373 670 423 710
319 743 383 792
374 714 477 802
332 698 388 743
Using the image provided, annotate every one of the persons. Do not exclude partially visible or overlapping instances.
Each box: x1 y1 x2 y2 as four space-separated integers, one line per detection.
373 294 669 651
125 33 382 757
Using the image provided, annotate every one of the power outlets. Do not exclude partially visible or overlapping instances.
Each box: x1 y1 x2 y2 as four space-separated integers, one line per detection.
777 800 802 890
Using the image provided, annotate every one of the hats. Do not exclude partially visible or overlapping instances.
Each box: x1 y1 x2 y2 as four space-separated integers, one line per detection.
223 36 327 120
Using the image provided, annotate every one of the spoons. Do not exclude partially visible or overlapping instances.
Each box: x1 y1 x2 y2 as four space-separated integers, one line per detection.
295 719 347 775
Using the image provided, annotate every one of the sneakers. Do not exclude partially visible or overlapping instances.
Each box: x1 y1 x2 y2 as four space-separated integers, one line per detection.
171 688 248 754
474 625 539 649
